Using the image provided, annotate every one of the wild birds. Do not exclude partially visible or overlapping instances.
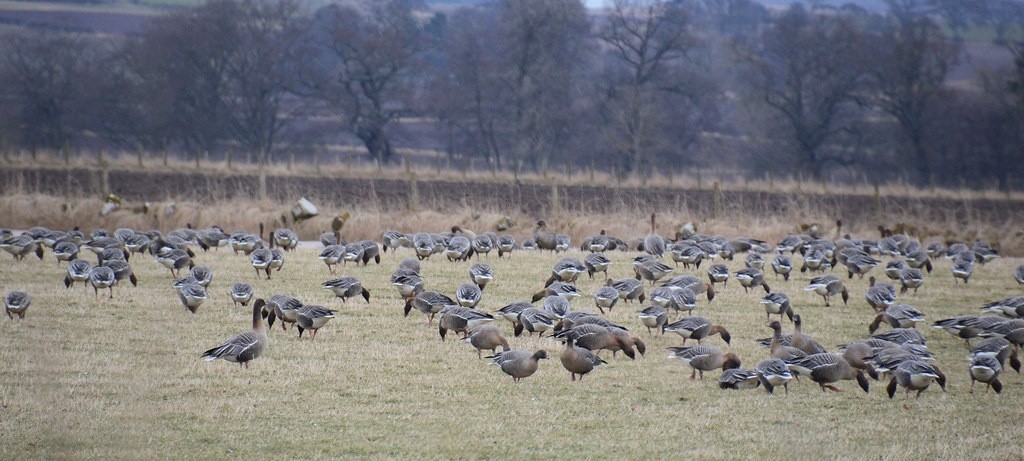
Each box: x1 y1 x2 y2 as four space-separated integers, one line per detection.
0 212 1024 401
